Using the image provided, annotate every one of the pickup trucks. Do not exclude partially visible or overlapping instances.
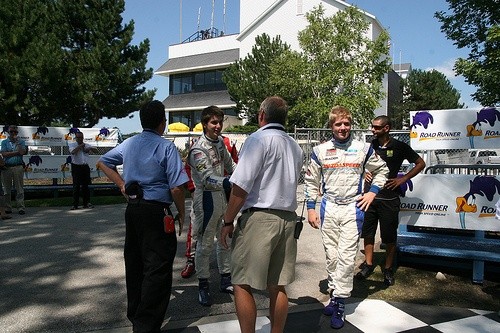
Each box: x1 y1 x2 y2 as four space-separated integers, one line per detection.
467 148 500 173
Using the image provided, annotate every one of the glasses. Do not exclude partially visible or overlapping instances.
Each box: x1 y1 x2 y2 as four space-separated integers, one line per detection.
8 130 19 135
372 125 385 130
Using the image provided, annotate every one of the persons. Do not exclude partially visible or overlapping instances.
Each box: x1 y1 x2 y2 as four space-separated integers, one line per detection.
0 127 27 214
361 116 426 286
305 105 390 328
181 106 238 306
98 100 189 333
221 97 303 333
69 132 97 209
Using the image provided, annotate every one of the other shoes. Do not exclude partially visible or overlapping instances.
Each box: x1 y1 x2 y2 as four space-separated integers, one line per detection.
199 282 212 306
323 298 335 316
384 267 395 286
18 208 25 215
70 205 78 210
5 210 13 214
330 302 346 329
181 263 195 278
220 284 235 295
355 264 374 280
83 202 94 209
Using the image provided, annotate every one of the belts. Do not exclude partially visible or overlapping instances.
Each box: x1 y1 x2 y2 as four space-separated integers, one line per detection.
242 207 268 214
73 164 89 168
5 163 23 168
323 193 362 205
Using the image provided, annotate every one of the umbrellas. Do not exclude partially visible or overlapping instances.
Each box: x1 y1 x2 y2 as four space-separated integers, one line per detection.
168 123 189 132
193 122 204 132
